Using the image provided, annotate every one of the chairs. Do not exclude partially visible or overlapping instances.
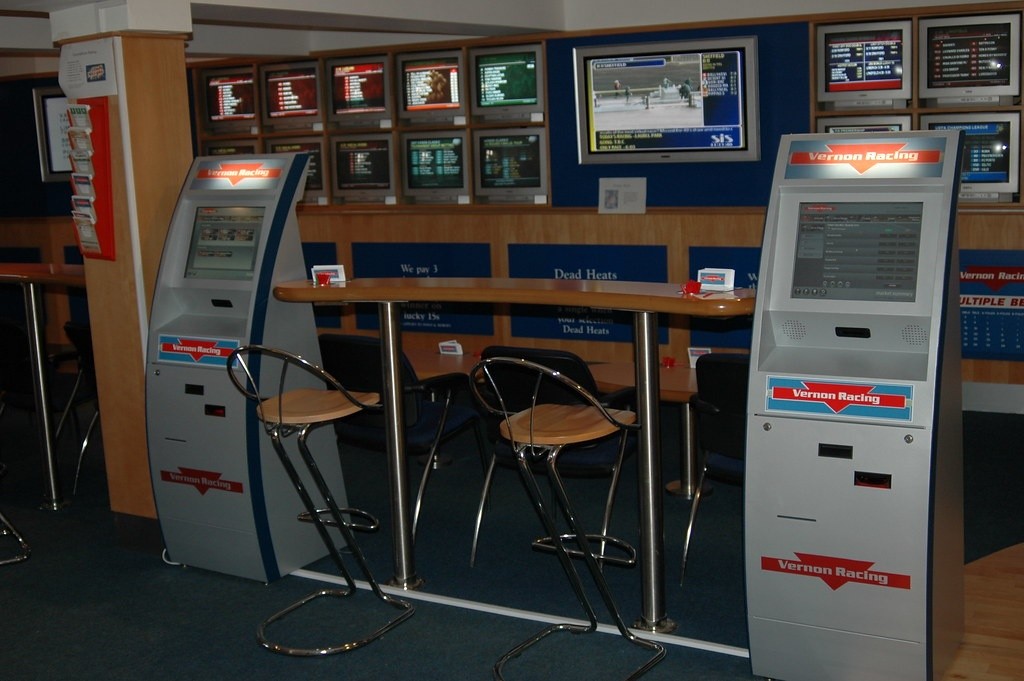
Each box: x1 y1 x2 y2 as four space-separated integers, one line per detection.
673 354 757 585
317 332 492 510
478 346 684 506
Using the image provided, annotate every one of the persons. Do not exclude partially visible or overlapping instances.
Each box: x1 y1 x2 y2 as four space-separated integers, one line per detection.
681 80 690 99
614 80 623 98
626 87 633 101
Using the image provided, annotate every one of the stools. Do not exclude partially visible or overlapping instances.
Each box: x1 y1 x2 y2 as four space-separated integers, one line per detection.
226 344 452 655
467 354 718 681
45 309 110 502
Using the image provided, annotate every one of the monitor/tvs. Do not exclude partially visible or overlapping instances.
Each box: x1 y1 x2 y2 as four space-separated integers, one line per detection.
573 35 762 163
792 202 923 303
184 44 548 280
816 13 1020 203
33 87 75 183
